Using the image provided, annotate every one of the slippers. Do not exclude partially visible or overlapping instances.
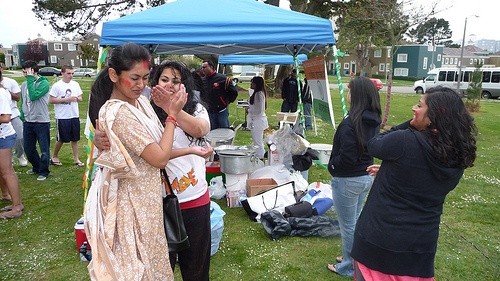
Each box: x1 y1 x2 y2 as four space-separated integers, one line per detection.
76 162 85 167
51 159 61 166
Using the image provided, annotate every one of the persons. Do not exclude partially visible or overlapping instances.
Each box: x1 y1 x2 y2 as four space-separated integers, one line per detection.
94 59 211 281
302 76 312 130
281 69 302 112
48 65 85 166
194 60 238 130
351 86 477 281
0 66 27 167
246 76 269 160
20 61 51 180
84 43 214 281
327 76 382 276
0 71 24 219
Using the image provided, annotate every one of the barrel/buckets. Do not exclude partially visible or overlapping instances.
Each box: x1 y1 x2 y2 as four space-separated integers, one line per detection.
224 173 248 192
74 216 92 262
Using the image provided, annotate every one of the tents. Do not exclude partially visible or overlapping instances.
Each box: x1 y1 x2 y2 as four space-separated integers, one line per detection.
83 0 347 199
216 53 308 75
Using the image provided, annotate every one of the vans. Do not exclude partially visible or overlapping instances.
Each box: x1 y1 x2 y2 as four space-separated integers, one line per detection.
413 66 500 100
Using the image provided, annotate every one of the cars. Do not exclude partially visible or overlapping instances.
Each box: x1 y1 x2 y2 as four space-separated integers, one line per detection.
72 67 96 78
227 71 263 84
37 67 62 76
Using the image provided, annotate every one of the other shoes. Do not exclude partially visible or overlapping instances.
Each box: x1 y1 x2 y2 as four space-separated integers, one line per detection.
327 263 337 273
0 195 11 200
18 154 27 167
27 169 36 174
1 205 24 211
37 174 46 181
0 211 23 218
336 256 342 262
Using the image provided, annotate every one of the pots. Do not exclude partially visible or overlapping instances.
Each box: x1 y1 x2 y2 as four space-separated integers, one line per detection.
216 149 257 174
311 143 332 165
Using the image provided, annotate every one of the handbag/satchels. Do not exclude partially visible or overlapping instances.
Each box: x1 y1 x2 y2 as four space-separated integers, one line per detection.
159 168 190 252
241 181 297 223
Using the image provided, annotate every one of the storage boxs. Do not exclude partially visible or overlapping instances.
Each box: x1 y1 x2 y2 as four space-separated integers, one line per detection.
246 178 279 197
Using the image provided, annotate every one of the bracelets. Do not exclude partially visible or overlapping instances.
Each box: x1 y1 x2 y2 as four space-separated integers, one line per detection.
166 117 176 124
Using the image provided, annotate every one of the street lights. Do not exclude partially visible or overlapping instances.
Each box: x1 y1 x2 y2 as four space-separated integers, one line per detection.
456 14 480 94
